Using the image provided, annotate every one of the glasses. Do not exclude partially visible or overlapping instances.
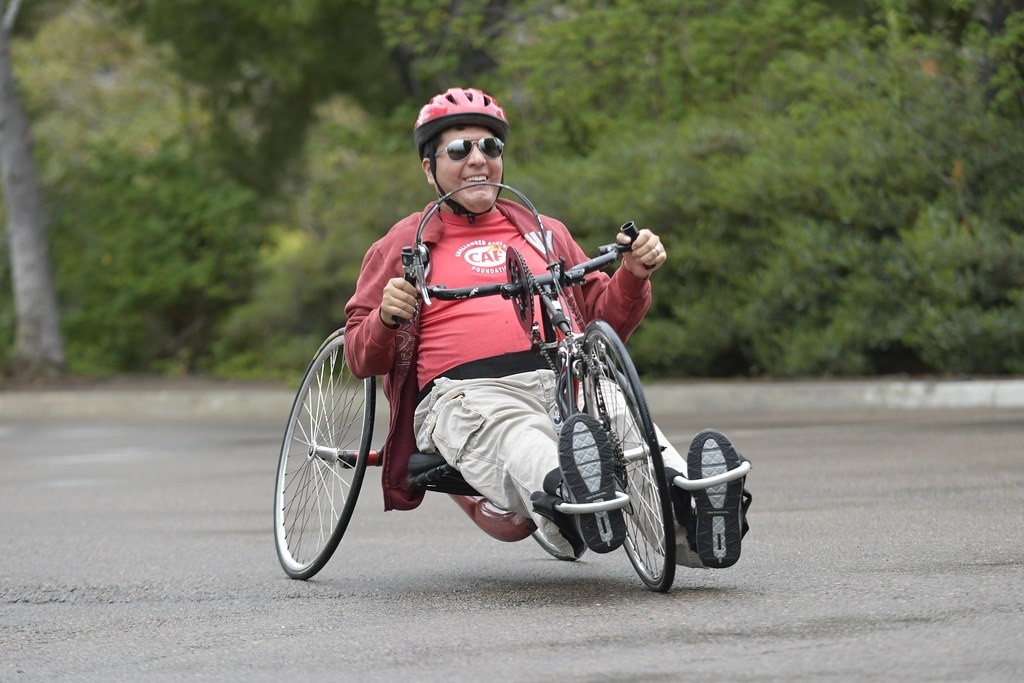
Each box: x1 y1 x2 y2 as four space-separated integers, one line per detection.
435 137 506 162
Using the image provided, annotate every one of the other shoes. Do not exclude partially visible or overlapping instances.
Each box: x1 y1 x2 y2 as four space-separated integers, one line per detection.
686 428 744 569
559 411 625 554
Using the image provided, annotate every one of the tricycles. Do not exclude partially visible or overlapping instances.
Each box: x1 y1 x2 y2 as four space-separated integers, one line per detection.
272 179 750 593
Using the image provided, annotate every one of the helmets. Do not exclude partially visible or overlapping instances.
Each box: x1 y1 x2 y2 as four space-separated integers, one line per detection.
414 87 510 161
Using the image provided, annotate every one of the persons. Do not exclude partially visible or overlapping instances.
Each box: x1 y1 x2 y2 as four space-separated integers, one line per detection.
342 86 747 570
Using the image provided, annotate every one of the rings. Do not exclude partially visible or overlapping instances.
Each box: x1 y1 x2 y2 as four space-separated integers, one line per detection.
655 246 661 254
